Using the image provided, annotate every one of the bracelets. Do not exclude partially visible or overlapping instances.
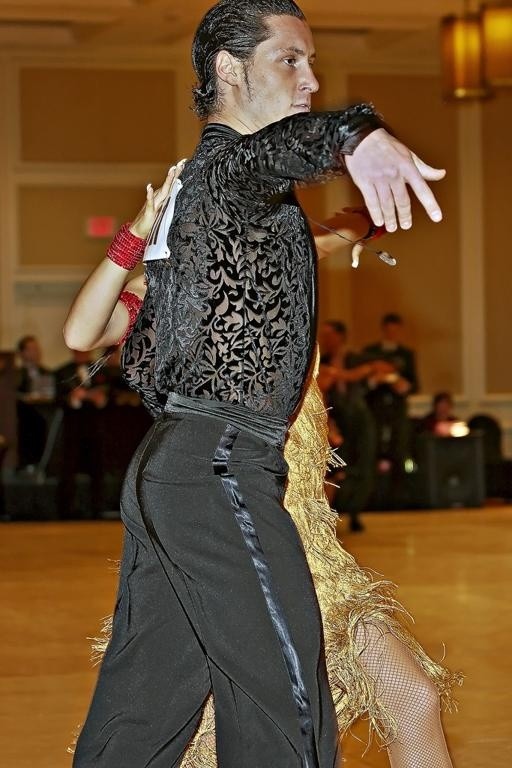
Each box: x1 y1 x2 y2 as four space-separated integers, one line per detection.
106 222 147 271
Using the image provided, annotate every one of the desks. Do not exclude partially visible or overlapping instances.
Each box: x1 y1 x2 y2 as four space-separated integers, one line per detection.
18 400 153 514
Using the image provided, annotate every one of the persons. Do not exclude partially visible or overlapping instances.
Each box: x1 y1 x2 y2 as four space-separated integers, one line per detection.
66 154 462 767
13 335 150 520
314 309 465 536
72 0 449 767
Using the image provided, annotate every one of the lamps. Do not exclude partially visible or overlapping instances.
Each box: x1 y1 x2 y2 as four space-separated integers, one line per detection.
439 0 512 104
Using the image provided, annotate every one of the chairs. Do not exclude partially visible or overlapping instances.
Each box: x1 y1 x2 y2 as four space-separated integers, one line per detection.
468 413 512 500
